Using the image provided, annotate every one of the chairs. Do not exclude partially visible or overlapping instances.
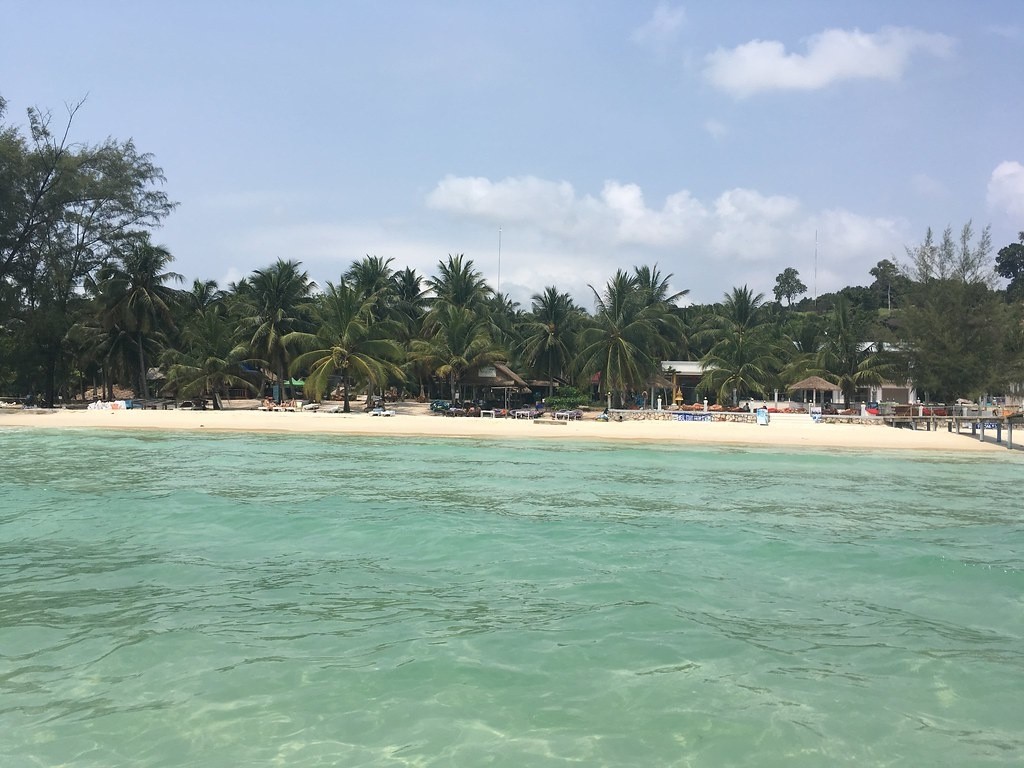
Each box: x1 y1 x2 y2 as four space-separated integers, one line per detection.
433 405 750 422
381 410 395 417
369 412 380 417
256 403 341 412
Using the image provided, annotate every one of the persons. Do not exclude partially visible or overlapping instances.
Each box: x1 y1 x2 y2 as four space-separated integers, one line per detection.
744 402 767 412
454 390 460 402
263 397 297 408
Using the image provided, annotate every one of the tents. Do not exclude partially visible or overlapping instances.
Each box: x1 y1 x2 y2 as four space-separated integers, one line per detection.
283 377 305 387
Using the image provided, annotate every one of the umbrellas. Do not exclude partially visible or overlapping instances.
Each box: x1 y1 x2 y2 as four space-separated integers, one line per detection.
641 373 677 410
788 376 842 407
